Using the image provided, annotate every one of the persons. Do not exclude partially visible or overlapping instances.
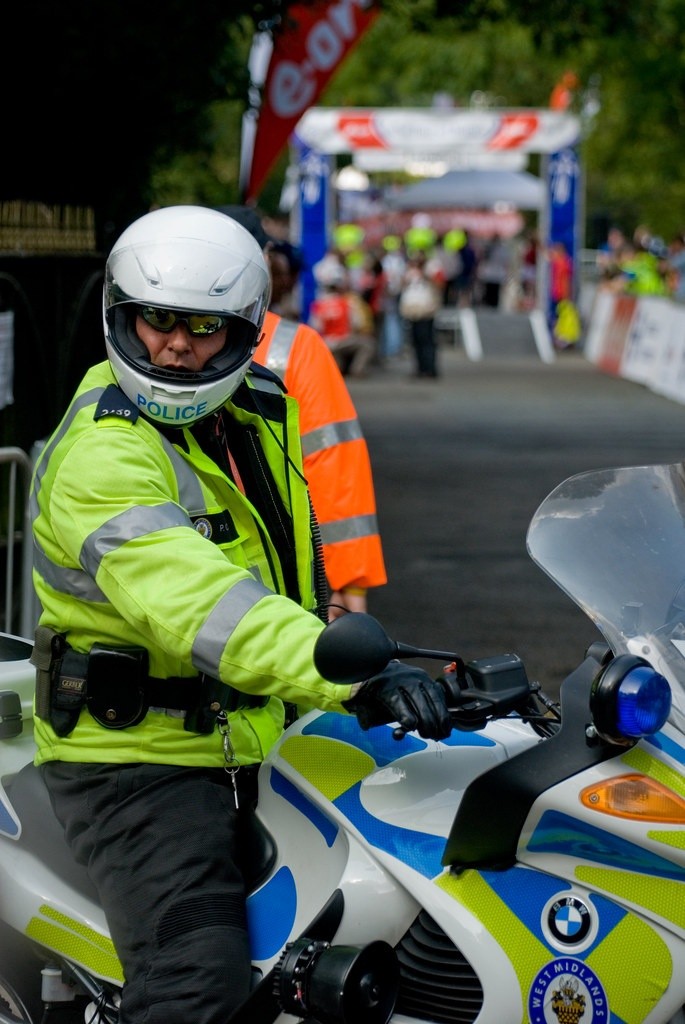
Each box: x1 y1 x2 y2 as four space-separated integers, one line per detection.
242 209 684 379
26 204 452 1024
244 309 388 621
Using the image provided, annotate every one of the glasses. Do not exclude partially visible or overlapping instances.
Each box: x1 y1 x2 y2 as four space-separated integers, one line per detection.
137 305 227 337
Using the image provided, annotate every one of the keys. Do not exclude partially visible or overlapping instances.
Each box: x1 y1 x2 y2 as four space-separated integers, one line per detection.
215 715 249 810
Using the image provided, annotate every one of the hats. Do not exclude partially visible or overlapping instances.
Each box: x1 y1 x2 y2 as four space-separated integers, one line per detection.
216 206 268 251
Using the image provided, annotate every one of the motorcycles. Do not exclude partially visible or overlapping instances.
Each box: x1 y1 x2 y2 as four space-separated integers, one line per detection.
0 463 685 1024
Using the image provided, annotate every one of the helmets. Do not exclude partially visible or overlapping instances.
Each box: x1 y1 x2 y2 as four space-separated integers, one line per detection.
103 205 272 429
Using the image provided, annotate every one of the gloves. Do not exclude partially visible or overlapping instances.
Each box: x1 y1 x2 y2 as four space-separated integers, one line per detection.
356 663 452 741
